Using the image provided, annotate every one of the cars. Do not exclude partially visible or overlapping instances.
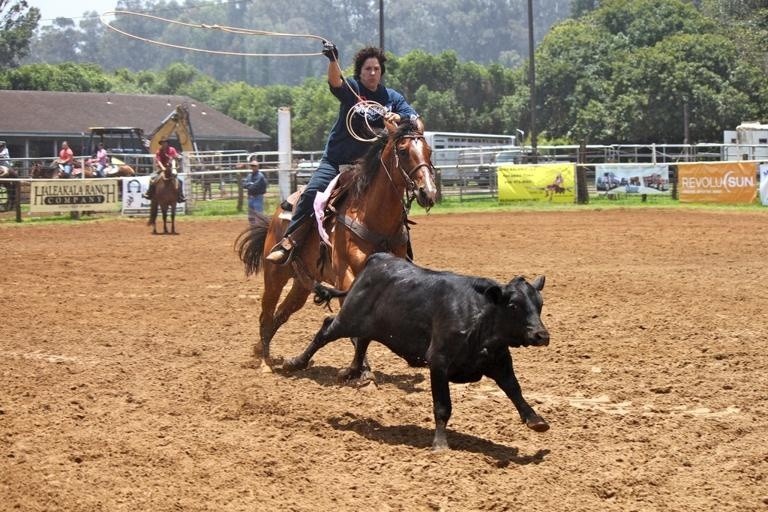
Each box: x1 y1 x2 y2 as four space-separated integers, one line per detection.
474 148 555 188
294 162 323 181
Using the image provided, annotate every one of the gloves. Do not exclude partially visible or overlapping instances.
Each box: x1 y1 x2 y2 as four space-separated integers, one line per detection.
321 39 338 61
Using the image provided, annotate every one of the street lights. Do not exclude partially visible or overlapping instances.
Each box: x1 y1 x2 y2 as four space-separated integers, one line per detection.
681 94 691 160
514 126 527 149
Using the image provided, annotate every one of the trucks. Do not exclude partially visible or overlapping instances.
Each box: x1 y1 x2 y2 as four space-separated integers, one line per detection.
598 170 620 192
720 121 768 177
643 173 669 192
621 175 629 186
630 177 639 183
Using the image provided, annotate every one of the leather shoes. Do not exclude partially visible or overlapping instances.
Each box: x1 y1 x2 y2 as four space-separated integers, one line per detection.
266 249 297 263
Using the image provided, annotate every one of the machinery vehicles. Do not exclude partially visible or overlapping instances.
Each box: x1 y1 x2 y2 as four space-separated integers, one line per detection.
88 105 206 198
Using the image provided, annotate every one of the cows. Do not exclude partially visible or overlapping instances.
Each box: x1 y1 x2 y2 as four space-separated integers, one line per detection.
282 250 551 451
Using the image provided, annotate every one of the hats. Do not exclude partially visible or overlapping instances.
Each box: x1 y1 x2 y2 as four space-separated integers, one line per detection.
250 160 258 165
158 136 169 145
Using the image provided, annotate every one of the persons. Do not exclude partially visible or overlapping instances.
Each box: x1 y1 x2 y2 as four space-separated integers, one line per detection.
202 169 212 200
244 160 267 225
0 141 10 165
59 142 73 174
267 41 423 263
142 135 187 203
89 142 108 177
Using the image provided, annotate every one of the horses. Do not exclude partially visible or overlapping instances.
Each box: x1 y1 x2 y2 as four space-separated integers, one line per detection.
76 158 135 177
0 164 18 212
232 115 438 384
147 154 179 235
30 161 92 217
